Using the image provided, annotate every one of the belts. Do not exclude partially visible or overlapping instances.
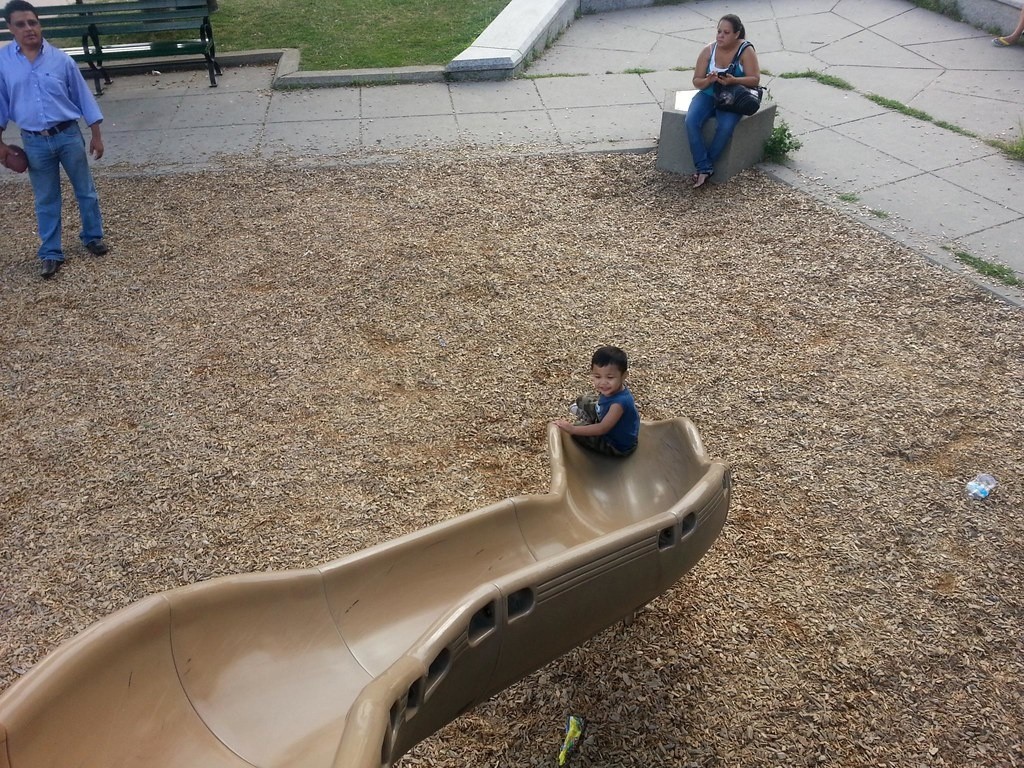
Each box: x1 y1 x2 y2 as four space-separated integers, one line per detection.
24 121 70 136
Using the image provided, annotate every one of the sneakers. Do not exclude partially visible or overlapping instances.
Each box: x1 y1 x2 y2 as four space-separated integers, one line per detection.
40 260 62 277
88 240 108 255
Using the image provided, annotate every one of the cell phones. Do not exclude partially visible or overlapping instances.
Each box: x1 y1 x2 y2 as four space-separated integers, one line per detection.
718 72 726 77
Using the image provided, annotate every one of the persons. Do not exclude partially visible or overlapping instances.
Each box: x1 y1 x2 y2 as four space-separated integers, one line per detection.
685 14 760 187
553 347 640 459
991 6 1024 46
0 0 111 278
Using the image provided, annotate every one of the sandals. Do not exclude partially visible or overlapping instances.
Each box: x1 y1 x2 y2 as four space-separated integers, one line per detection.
693 171 714 189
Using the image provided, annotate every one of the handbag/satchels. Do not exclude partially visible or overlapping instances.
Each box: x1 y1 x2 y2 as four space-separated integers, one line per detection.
714 41 767 116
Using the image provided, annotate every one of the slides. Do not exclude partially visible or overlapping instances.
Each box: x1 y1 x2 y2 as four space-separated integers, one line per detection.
0 416 731 768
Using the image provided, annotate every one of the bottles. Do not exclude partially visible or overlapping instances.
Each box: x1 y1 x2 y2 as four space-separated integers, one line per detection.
964 473 996 500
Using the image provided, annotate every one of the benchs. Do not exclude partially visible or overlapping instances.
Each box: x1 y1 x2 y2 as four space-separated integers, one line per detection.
0 0 224 96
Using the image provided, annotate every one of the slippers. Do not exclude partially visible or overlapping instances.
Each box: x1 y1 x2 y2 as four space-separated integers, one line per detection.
991 37 1010 47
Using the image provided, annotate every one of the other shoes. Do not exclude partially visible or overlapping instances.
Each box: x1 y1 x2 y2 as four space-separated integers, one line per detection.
569 404 590 423
